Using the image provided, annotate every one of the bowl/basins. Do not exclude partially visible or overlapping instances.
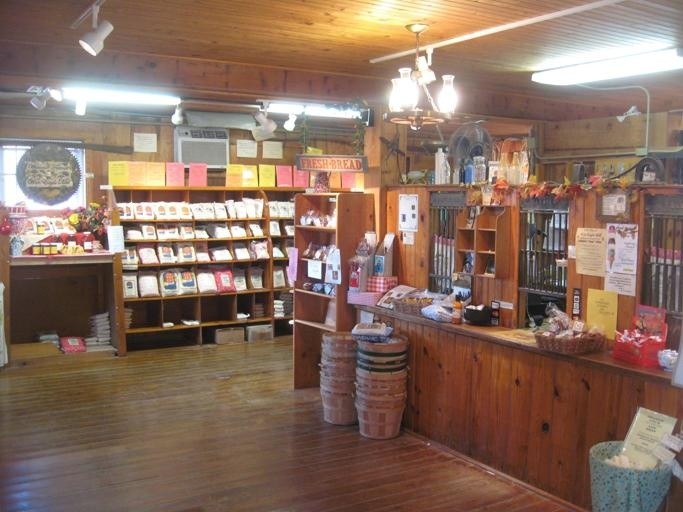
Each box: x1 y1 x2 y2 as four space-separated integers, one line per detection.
657 350 679 372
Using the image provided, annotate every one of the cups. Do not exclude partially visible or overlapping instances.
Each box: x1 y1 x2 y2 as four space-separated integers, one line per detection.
480 185 494 206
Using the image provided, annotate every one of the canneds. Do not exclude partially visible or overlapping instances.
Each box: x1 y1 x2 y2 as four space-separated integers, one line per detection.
65 231 93 252
32 242 58 255
37 222 45 234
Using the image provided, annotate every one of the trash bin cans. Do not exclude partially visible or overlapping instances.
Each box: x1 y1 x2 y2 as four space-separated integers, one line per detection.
589 438 671 512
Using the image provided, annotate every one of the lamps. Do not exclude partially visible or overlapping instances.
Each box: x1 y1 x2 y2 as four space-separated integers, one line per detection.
25 84 305 143
381 23 460 132
70 0 114 57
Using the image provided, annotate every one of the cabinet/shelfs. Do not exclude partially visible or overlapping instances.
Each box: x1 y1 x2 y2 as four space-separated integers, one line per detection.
454 205 511 279
99 183 365 356
293 192 375 388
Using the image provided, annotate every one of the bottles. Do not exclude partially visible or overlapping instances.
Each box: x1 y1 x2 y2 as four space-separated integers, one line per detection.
37 223 45 235
0 215 12 235
435 147 529 184
81 231 93 252
349 272 359 292
67 233 76 252
451 294 462 325
32 243 58 255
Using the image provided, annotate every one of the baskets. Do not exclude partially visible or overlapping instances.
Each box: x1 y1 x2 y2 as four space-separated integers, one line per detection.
536 329 606 355
392 297 432 315
